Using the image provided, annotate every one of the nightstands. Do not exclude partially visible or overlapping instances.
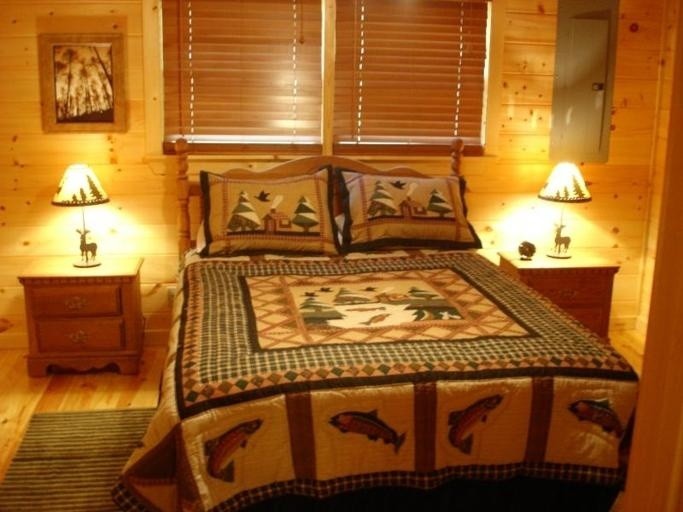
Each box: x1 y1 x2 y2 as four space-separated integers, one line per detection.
497 245 621 344
13 256 146 379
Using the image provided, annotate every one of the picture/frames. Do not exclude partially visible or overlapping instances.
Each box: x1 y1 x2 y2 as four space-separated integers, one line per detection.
39 29 130 133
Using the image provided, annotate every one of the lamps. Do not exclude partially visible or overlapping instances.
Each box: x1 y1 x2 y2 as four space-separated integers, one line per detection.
51 163 111 269
538 160 591 260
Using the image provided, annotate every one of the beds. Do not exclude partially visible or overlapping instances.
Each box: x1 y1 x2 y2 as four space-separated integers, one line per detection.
163 137 643 509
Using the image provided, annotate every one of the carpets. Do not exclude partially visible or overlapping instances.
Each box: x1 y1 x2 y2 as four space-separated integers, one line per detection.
4 401 176 512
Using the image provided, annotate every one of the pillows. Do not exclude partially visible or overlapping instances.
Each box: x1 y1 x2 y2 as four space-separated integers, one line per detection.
190 160 484 259
